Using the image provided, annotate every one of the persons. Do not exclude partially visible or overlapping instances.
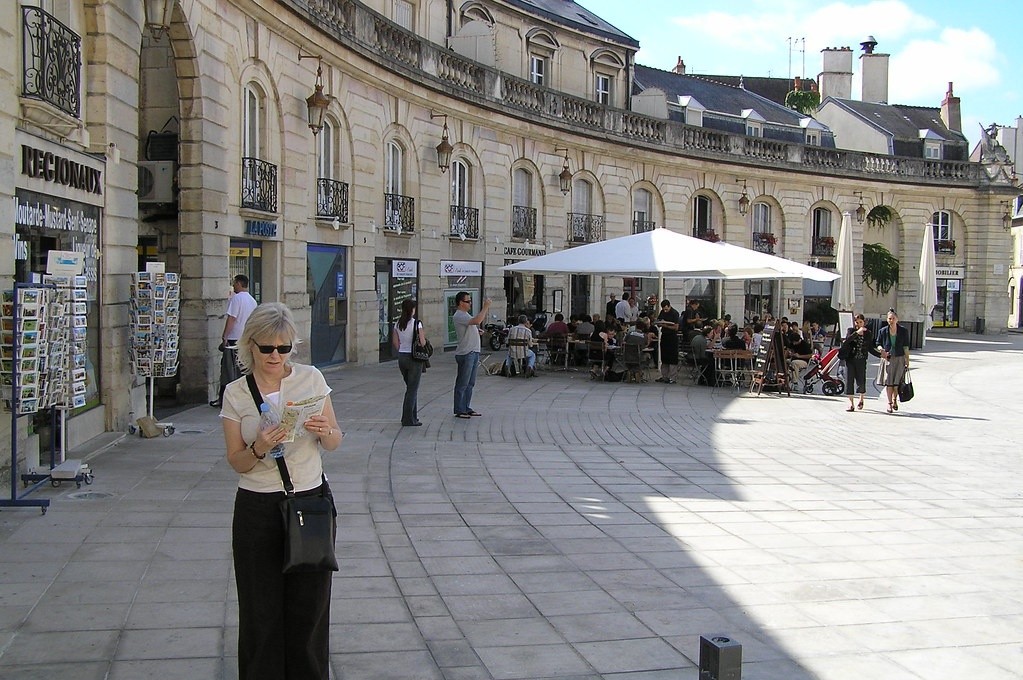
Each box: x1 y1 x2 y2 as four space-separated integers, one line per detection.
392 299 426 427
218 301 342 680
875 307 909 413
504 292 827 392
209 274 258 408
846 313 888 411
655 300 680 384
452 292 492 418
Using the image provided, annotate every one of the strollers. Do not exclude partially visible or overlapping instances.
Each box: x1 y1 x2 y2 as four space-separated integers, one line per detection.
802 348 845 396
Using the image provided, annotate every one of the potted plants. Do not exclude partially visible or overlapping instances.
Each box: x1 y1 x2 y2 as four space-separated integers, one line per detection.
936 240 953 252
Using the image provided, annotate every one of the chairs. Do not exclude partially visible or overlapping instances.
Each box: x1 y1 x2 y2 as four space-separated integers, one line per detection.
505 325 825 396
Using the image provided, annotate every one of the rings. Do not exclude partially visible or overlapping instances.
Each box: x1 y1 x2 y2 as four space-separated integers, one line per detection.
319 428 322 432
272 440 277 443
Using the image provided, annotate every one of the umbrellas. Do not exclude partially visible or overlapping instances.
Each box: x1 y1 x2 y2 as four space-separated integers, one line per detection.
830 211 856 312
918 222 938 347
496 225 843 373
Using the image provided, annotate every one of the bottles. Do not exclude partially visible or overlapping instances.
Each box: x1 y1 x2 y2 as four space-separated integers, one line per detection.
259 403 287 458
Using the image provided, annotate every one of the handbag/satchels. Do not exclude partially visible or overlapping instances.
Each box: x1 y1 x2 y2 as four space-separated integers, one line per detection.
411 318 429 361
898 368 914 402
282 493 339 573
837 327 858 360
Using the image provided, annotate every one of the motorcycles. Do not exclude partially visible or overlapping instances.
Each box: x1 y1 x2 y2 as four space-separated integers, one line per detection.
487 319 513 351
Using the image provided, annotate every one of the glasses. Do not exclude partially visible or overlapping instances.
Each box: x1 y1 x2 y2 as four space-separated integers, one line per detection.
253 339 292 354
462 300 471 303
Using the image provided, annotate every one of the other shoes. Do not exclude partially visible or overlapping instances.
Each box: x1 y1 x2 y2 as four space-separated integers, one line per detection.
655 377 677 384
794 383 799 390
846 405 853 411
453 411 482 418
400 419 422 426
504 366 511 377
525 366 532 378
209 399 220 407
887 399 898 413
857 401 863 410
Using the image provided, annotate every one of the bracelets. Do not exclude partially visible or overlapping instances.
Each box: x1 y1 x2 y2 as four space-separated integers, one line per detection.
250 440 266 461
329 428 333 437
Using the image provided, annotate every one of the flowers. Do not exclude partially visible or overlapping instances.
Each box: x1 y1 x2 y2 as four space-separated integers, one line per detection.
700 230 721 244
761 232 778 247
819 237 835 245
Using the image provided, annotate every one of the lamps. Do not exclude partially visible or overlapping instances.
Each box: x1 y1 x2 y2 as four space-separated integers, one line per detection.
736 179 750 217
538 240 553 249
139 160 177 203
42 122 91 149
481 235 500 243
89 141 121 165
429 111 454 176
999 199 1013 232
310 215 340 230
854 190 866 225
378 224 401 236
443 232 466 242
555 145 573 196
513 237 529 247
297 53 332 136
145 0 174 43
415 228 437 239
350 220 376 233
